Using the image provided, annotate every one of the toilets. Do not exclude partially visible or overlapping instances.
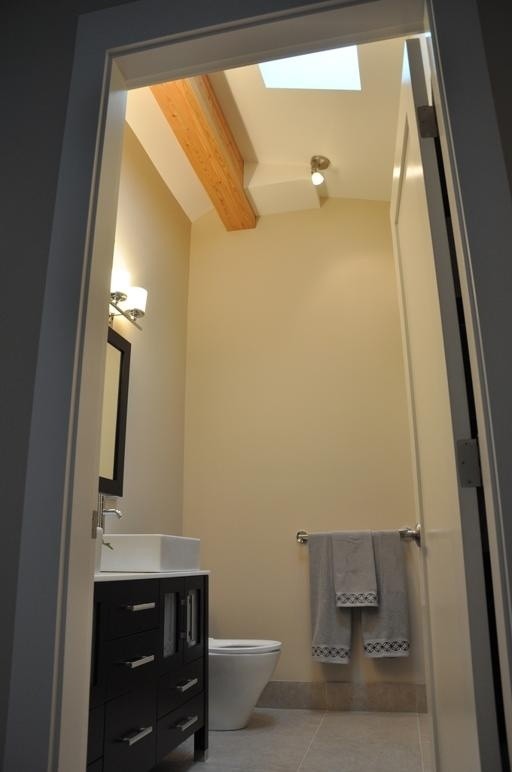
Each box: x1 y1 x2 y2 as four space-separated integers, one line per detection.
208 636 283 731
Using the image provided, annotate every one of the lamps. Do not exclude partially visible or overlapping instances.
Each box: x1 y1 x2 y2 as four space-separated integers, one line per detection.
108 266 150 332
309 155 330 187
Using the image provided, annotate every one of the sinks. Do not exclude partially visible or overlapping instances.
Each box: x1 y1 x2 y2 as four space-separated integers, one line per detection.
100 531 202 573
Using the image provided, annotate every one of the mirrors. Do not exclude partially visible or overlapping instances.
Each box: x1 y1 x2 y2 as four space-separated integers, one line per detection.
97 327 131 496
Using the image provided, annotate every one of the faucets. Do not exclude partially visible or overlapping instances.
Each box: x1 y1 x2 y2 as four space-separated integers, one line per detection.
96 494 125 535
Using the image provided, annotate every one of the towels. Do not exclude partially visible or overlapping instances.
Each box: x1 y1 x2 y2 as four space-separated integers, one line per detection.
307 528 413 667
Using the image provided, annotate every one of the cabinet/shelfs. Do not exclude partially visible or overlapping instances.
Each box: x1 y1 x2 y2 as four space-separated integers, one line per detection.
86 575 209 771
